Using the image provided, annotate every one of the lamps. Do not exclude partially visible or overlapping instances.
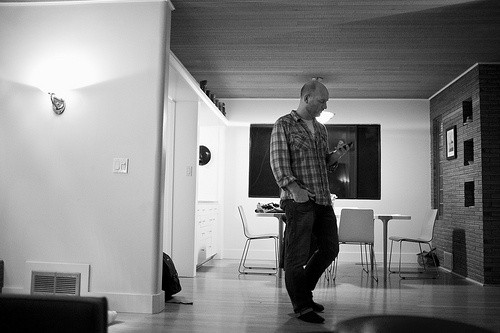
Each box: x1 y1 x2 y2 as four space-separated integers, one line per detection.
312 77 334 124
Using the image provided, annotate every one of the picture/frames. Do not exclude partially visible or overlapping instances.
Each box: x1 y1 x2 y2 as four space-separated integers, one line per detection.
446 125 457 160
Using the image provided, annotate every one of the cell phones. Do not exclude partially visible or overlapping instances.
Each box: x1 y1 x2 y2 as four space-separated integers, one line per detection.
339 142 353 151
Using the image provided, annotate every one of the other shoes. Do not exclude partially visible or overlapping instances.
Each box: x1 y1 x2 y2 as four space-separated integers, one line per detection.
296 310 325 324
309 300 324 312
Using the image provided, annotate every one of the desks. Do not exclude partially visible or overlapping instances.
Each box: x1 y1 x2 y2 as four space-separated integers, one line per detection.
257 213 411 280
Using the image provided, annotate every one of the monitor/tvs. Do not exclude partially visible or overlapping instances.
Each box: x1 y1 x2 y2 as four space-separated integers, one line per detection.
249 124 381 200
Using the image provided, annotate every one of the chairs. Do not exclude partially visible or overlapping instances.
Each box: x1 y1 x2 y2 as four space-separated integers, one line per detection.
389 209 439 280
238 205 279 275
325 209 378 282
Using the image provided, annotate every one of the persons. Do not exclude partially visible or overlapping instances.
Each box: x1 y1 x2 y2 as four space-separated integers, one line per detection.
269 82 348 324
